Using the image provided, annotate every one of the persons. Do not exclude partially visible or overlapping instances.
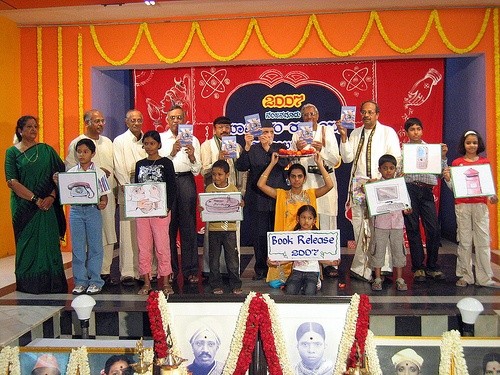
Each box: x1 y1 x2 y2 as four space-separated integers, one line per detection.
391 348 424 375
292 322 334 375
31 355 61 375
482 352 500 375
53 101 448 296
443 130 500 288
5 115 69 294
186 325 224 375
100 355 135 375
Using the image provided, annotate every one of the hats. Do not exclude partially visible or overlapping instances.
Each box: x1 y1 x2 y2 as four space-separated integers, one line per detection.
261 120 272 127
391 348 424 370
31 354 62 374
213 116 232 124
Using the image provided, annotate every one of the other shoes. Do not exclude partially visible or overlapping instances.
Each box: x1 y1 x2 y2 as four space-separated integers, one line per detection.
122 277 135 287
396 278 407 291
186 275 199 284
372 278 382 291
101 274 118 286
426 270 446 281
413 270 426 282
325 266 339 277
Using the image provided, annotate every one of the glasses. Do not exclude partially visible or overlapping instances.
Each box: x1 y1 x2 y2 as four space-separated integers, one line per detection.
303 112 316 116
360 111 376 115
168 116 183 119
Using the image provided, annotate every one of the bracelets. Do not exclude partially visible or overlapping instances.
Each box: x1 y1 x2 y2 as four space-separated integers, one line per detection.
49 195 56 200
31 195 38 204
261 175 268 178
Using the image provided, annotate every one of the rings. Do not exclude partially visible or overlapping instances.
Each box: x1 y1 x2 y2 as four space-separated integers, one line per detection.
42 205 49 210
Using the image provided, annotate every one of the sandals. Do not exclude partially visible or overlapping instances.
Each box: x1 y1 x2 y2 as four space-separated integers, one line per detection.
213 288 224 295
138 284 152 295
232 288 243 296
162 284 174 295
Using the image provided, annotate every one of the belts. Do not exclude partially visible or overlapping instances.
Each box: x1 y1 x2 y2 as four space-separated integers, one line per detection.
408 182 433 189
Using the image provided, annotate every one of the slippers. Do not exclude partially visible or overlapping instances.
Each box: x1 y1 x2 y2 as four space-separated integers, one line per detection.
456 278 467 287
475 280 500 288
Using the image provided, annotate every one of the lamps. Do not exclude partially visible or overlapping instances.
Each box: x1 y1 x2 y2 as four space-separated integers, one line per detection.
71 295 97 328
456 297 485 332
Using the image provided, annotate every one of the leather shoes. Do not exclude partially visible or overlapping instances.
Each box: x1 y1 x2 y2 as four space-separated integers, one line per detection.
253 272 267 280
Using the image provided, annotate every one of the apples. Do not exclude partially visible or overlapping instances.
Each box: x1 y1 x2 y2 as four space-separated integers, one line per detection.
278 148 314 155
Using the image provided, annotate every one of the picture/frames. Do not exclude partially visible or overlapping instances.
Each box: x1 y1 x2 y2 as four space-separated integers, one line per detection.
401 143 442 175
198 191 244 223
151 293 258 375
57 171 100 205
363 176 412 218
453 337 500 375
450 162 498 199
78 346 153 375
257 293 369 375
123 182 168 218
366 335 452 375
6 346 78 375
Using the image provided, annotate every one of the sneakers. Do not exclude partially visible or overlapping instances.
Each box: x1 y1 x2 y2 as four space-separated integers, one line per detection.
72 284 102 295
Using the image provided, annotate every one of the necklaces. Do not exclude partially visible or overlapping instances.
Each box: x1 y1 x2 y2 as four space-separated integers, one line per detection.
147 155 159 166
20 140 38 162
